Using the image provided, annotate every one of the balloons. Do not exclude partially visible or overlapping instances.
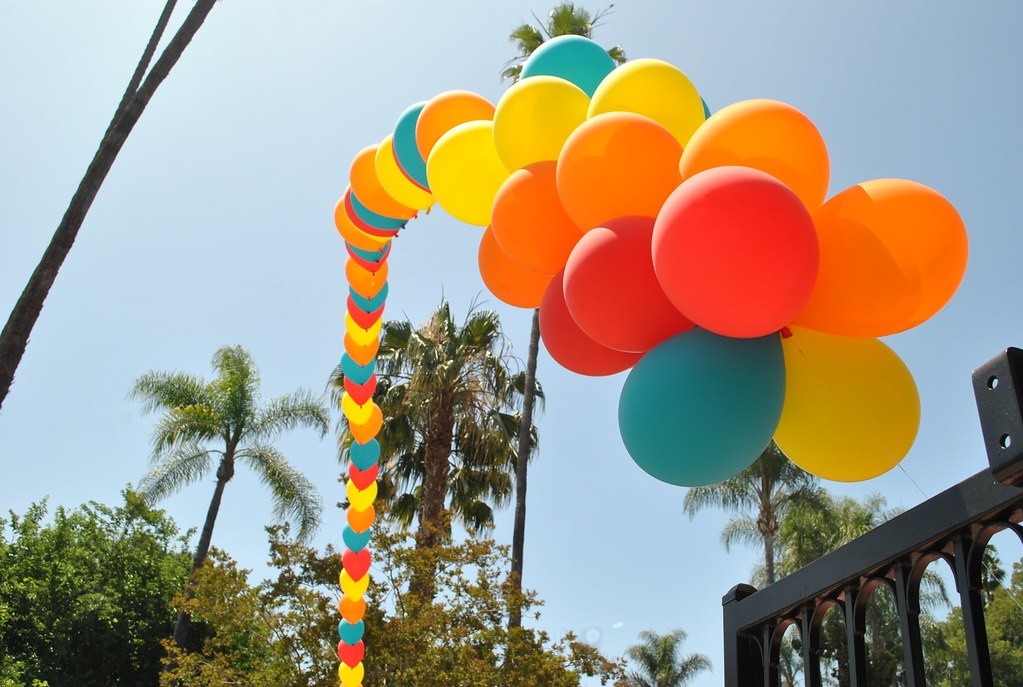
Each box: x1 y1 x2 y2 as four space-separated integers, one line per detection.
332 33 970 687
